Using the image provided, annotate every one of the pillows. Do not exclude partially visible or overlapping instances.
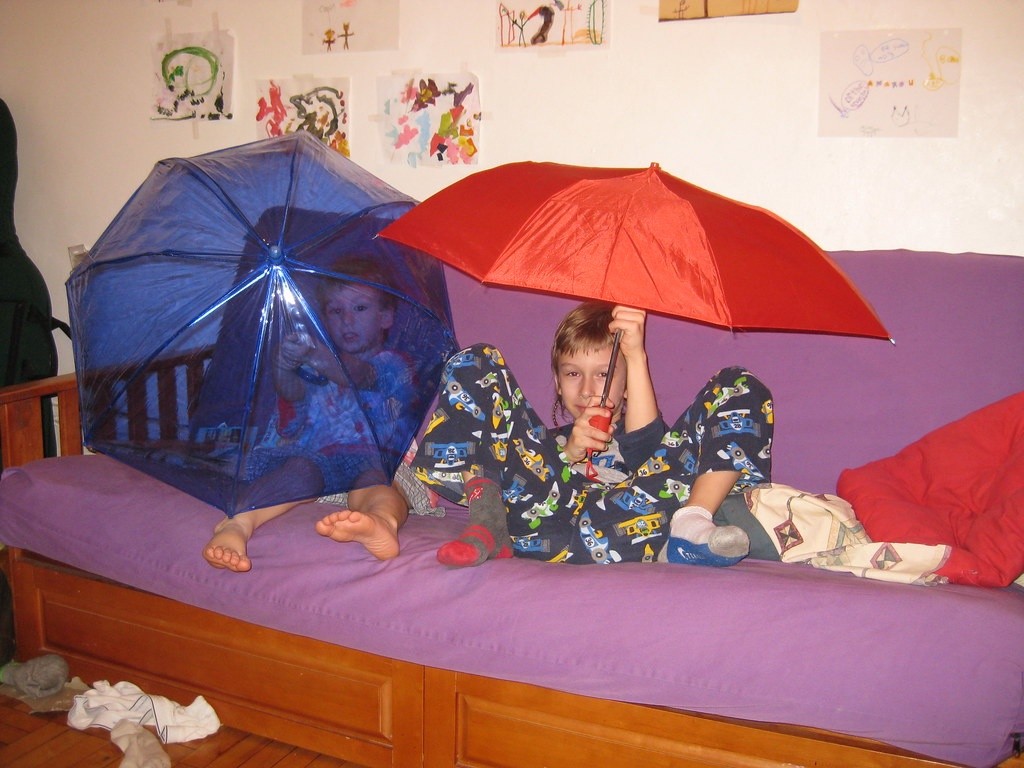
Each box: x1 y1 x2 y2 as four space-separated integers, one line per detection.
836 389 1023 588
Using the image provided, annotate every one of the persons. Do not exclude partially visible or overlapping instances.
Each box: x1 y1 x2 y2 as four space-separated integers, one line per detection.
201 250 445 572
409 301 774 567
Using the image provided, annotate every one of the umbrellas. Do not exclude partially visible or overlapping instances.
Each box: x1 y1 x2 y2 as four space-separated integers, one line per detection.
370 159 896 478
62 130 456 521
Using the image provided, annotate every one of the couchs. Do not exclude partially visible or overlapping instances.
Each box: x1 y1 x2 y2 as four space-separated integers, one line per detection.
0 205 1024 768
